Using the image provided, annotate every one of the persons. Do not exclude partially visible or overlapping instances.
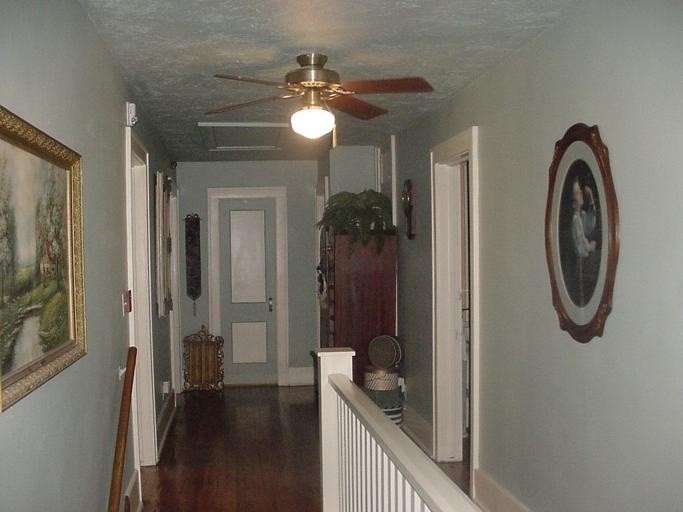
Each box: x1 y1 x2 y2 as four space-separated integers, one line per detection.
563 175 600 302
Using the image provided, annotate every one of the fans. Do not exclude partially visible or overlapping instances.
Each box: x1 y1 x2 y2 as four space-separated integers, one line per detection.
205 54 435 122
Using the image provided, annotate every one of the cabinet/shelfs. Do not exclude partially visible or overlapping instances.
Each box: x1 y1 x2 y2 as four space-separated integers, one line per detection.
319 227 398 385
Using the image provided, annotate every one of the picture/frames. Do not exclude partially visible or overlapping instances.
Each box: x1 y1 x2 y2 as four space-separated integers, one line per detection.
542 122 619 344
1 104 88 414
